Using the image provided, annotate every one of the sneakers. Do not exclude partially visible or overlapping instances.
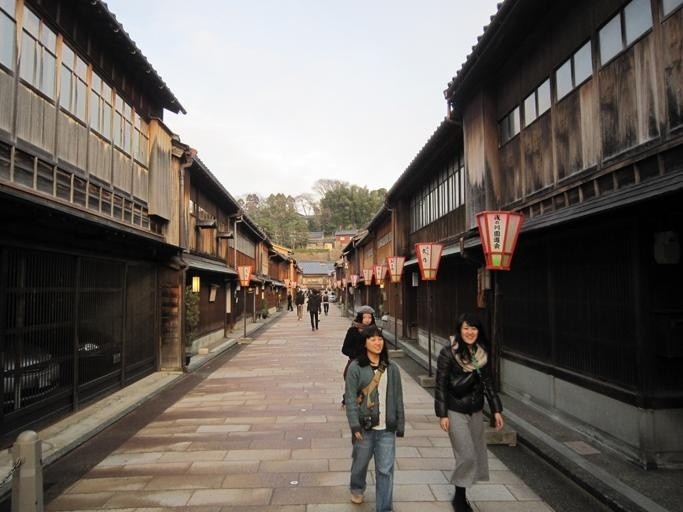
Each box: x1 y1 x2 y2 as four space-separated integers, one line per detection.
350 493 363 503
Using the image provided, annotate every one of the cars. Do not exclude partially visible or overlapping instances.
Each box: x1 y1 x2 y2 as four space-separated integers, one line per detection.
0 324 119 408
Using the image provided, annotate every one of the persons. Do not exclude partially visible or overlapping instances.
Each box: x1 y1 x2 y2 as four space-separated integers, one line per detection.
436 312 503 512
287 288 329 331
342 305 404 512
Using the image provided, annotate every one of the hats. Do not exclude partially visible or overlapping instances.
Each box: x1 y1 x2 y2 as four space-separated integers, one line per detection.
355 305 375 313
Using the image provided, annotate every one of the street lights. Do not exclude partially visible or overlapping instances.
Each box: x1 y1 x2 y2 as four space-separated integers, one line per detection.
477 211 525 446
333 274 358 319
237 265 252 344
414 242 446 387
362 256 406 358
